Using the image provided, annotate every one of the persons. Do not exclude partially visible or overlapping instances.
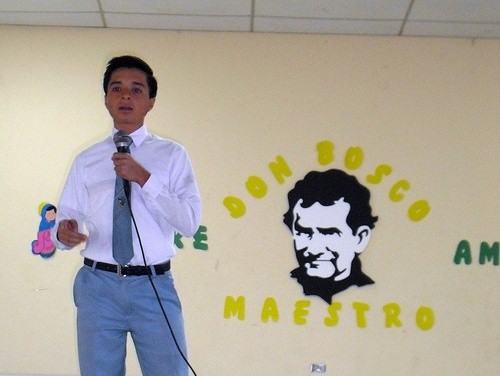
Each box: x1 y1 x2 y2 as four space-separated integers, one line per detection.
50 55 202 376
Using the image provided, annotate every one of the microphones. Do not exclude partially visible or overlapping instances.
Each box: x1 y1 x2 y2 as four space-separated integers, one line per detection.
113 136 133 196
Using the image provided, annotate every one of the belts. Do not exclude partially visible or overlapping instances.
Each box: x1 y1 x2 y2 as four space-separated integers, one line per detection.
84 257 170 276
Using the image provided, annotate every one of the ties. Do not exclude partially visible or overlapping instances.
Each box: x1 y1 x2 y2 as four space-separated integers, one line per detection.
113 136 135 268
